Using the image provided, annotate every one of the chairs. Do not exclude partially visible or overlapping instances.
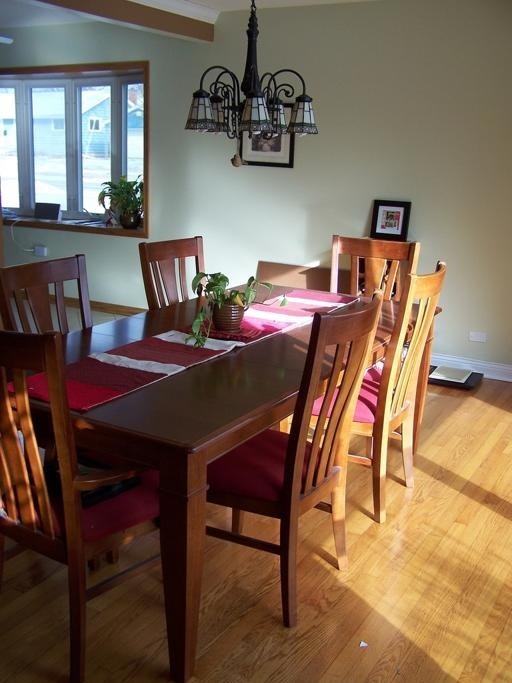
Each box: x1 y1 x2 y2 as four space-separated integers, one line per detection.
0 233 449 683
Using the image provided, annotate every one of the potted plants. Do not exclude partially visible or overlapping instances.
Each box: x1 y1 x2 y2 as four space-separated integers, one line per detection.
96 174 145 231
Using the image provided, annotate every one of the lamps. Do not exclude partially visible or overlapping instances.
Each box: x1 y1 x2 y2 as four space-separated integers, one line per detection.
184 0 319 141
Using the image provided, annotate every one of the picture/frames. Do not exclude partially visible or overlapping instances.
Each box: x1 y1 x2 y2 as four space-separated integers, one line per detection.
369 199 412 243
238 102 297 169
